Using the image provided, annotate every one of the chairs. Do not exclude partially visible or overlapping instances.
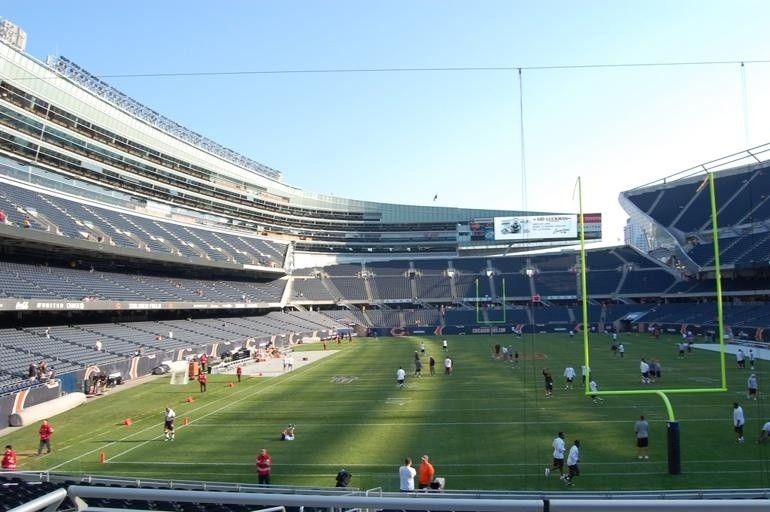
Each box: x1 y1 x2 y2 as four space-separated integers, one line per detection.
0 476 351 512
618 159 770 344
292 241 678 334
0 166 287 398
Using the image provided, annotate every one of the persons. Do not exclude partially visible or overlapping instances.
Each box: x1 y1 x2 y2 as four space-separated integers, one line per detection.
192 329 353 392
541 363 605 405
493 341 522 370
544 429 581 488
44 325 53 340
91 370 111 396
0 444 18 472
730 347 770 443
335 468 353 488
162 406 177 443
254 447 275 488
93 338 105 353
35 419 54 456
0 208 32 230
602 326 720 385
393 338 453 390
80 294 100 302
635 415 650 459
27 361 59 382
399 454 445 491
280 422 298 443
